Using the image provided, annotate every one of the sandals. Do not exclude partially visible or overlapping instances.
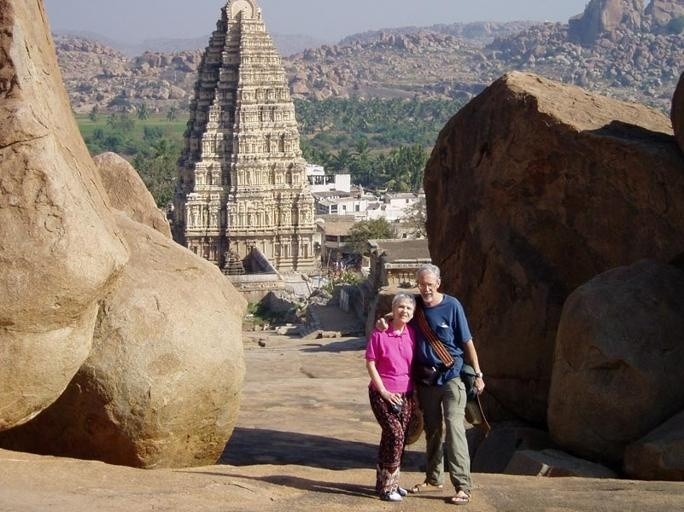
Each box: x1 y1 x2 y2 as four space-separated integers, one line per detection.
450 490 470 505
407 480 442 494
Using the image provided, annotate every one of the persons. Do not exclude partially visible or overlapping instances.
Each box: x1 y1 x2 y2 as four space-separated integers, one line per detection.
374 263 487 506
359 292 420 504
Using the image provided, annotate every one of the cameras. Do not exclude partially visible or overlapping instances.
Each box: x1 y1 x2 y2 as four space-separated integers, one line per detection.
390 397 403 412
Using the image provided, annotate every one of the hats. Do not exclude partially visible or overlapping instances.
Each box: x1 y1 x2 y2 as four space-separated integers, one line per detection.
404 409 424 445
464 393 492 440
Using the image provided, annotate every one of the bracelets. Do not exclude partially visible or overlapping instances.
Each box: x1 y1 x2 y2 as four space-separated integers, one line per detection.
475 370 483 378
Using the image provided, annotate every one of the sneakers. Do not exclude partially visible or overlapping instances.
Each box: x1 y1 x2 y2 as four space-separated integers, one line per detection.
388 492 402 502
396 487 406 497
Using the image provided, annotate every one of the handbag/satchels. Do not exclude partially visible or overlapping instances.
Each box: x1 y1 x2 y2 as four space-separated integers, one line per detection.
460 364 477 400
